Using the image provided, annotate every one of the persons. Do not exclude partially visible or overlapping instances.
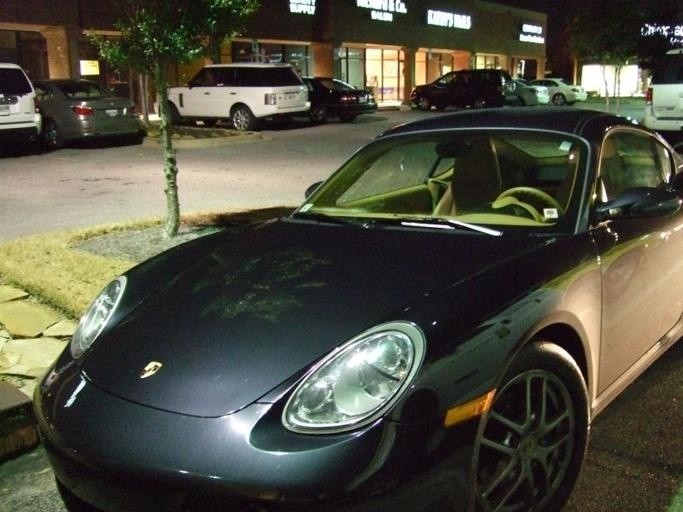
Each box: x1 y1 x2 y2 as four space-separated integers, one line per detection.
425 140 508 218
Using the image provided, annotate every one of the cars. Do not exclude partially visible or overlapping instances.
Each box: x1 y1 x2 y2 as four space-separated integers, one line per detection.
529 77 588 107
503 78 550 107
32 78 145 149
271 76 379 124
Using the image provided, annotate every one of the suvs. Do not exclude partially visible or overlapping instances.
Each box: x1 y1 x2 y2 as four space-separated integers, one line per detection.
411 68 519 112
154 61 313 130
0 61 44 152
642 47 682 140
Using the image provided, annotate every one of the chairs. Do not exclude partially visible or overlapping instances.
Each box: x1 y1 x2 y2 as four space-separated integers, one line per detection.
431 138 656 228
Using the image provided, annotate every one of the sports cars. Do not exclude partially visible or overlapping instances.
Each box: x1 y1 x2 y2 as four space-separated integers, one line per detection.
28 108 682 511
